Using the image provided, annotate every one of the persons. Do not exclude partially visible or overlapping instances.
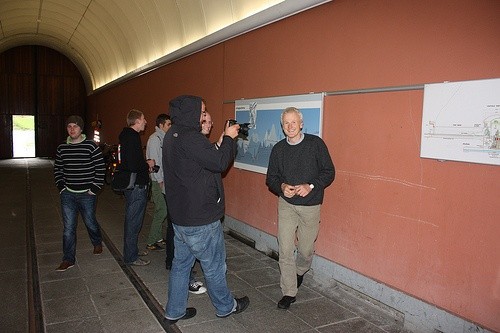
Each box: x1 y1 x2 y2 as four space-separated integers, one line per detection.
119 109 156 266
265 106 336 311
146 94 250 325
55 116 106 273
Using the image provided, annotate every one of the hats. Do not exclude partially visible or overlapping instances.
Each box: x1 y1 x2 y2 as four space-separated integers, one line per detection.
65 115 84 129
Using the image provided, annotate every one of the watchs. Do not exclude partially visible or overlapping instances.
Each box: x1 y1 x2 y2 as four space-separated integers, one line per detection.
308 182 315 191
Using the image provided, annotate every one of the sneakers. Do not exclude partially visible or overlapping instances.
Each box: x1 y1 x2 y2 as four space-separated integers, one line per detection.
146 243 165 252
157 238 167 245
188 280 208 294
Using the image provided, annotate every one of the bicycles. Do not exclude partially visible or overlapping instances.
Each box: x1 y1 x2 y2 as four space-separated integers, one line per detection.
99 141 118 185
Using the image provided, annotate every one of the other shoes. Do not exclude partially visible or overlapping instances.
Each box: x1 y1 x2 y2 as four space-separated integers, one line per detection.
216 296 249 318
297 270 309 288
93 243 103 256
278 295 296 311
55 261 75 272
164 307 196 325
129 256 150 266
138 248 148 256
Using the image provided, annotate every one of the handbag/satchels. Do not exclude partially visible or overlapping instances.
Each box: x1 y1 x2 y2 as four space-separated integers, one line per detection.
111 170 137 191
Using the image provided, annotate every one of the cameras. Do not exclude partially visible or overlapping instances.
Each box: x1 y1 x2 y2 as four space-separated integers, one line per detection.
148 159 161 173
224 120 250 141
